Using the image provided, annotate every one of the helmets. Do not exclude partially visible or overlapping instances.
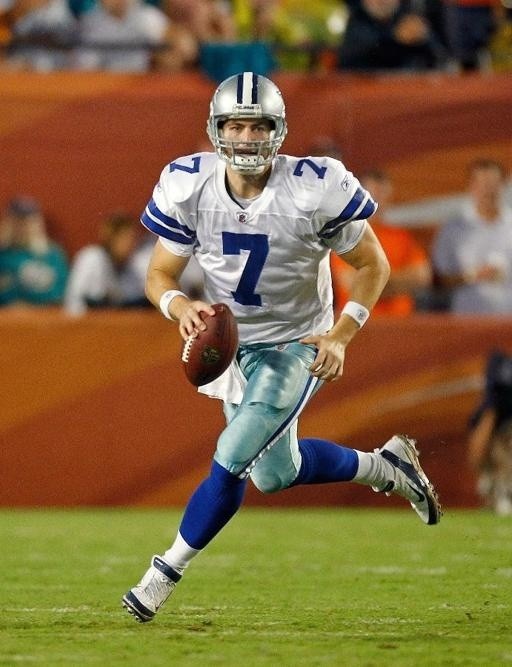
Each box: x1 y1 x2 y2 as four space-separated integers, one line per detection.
205 72 287 176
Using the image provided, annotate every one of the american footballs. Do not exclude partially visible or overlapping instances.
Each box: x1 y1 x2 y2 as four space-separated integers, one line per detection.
181 303 238 385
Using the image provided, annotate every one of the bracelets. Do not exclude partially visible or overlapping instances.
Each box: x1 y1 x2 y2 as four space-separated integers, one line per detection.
160 290 189 320
341 301 370 329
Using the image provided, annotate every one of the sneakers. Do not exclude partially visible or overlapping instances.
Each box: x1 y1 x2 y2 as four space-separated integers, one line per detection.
371 433 443 524
122 553 182 623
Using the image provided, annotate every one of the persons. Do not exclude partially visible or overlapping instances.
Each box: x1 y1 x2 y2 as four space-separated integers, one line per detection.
122 72 442 622
430 160 511 314
66 213 157 318
0 0 512 85
466 350 512 517
329 170 436 319
1 194 72 307
